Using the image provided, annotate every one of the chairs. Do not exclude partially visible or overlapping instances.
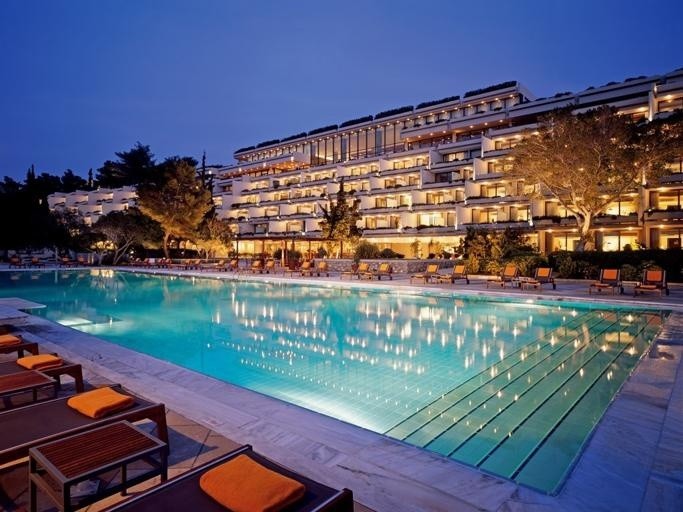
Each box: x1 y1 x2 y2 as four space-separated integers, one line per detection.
339 262 394 281
283 261 330 278
634 264 668 297
1 383 169 470
8 253 90 272
1 334 39 359
106 443 353 511
485 263 556 295
409 264 470 287
1 352 84 393
120 255 277 275
589 268 621 297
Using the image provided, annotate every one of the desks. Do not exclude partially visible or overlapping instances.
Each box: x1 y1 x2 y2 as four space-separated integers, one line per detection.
1 370 60 400
28 419 168 511
617 280 642 297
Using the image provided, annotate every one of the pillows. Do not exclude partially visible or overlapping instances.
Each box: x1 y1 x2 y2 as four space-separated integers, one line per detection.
199 453 305 512
67 386 134 418
17 354 64 370
1 335 21 346
